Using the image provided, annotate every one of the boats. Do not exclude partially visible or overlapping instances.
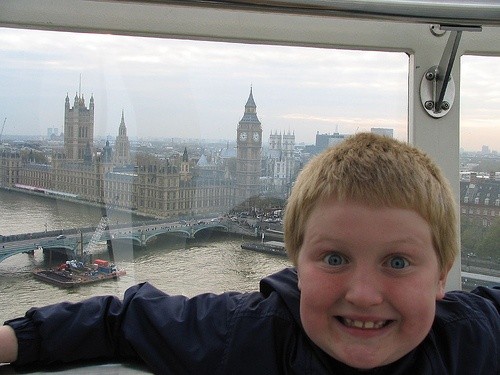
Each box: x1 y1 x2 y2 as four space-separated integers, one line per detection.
33 258 125 287
241 239 287 256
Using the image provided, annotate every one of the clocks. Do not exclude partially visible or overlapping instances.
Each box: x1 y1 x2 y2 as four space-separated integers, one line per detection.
252 132 259 142
239 132 247 142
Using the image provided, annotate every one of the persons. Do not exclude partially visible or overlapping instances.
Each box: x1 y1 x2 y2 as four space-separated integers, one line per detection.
1 132 500 375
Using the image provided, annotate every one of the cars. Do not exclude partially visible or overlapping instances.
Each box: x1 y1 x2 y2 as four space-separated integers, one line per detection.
57 235 65 240
176 211 248 228
